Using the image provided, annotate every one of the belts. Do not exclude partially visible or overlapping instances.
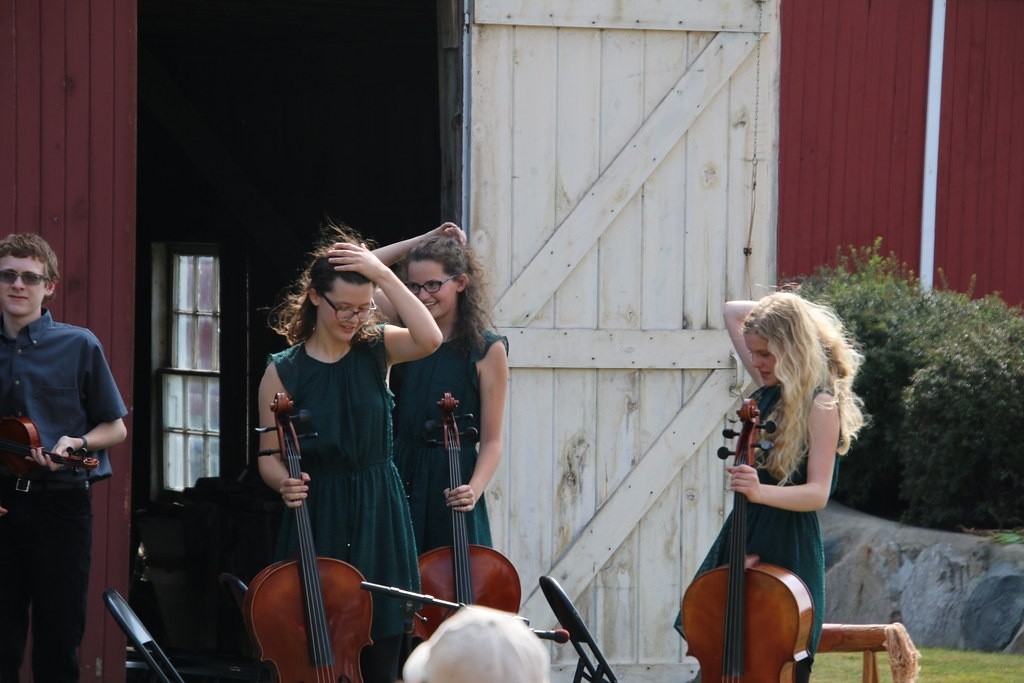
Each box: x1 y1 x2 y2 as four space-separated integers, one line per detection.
0 475 83 493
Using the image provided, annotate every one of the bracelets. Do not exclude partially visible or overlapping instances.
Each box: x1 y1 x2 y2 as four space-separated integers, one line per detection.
76 433 88 457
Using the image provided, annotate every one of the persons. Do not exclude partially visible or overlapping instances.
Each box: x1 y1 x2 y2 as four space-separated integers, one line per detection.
672 290 869 683
0 234 129 683
257 224 443 682
372 221 507 644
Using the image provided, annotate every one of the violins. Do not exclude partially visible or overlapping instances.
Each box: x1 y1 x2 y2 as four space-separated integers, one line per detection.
0 414 100 475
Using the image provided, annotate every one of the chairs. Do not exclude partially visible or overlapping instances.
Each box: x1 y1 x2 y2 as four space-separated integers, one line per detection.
538 575 617 683
102 587 184 683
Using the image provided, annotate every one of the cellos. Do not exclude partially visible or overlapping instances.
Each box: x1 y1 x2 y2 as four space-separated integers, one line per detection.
676 396 819 683
409 390 523 641
242 390 377 683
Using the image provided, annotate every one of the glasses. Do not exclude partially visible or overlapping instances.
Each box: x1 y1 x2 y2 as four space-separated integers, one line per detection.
405 274 456 294
0 270 49 286
316 288 377 322
